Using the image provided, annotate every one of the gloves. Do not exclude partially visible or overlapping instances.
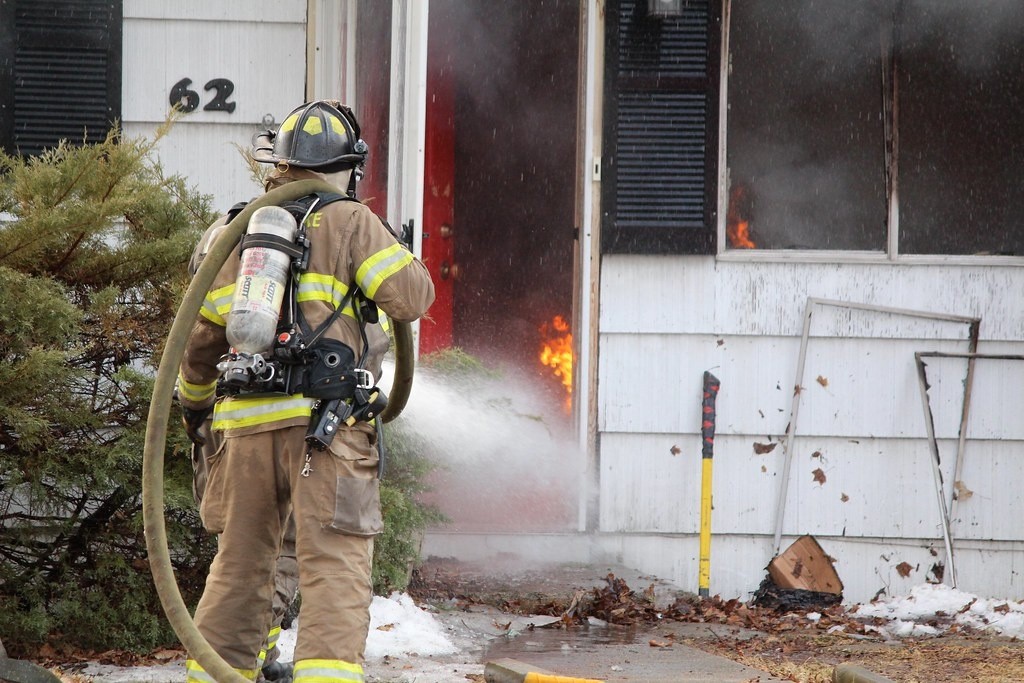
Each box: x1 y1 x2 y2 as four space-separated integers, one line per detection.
182 404 212 444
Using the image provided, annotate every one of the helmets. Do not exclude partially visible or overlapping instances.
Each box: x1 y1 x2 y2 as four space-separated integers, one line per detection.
250 99 366 167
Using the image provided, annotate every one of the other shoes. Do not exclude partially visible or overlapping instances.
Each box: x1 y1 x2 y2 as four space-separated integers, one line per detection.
252 660 296 683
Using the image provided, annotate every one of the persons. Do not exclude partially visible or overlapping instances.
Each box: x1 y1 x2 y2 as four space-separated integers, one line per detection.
173 100 436 683
